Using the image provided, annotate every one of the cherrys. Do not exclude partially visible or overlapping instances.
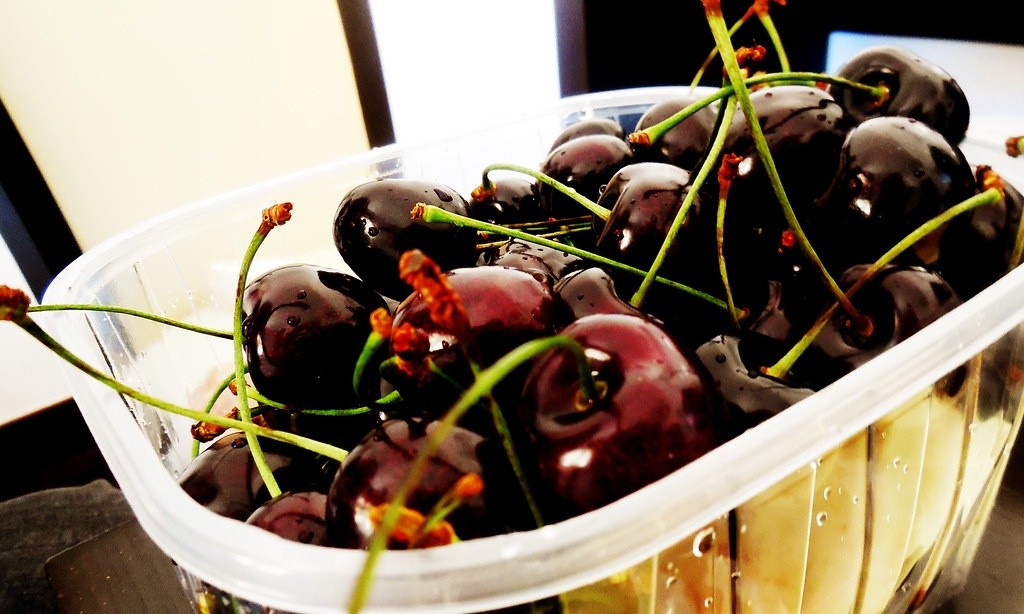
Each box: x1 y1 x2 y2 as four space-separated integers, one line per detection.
0 0 1024 614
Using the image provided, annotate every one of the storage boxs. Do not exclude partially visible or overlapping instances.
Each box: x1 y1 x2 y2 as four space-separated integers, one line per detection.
38 82 1024 614
